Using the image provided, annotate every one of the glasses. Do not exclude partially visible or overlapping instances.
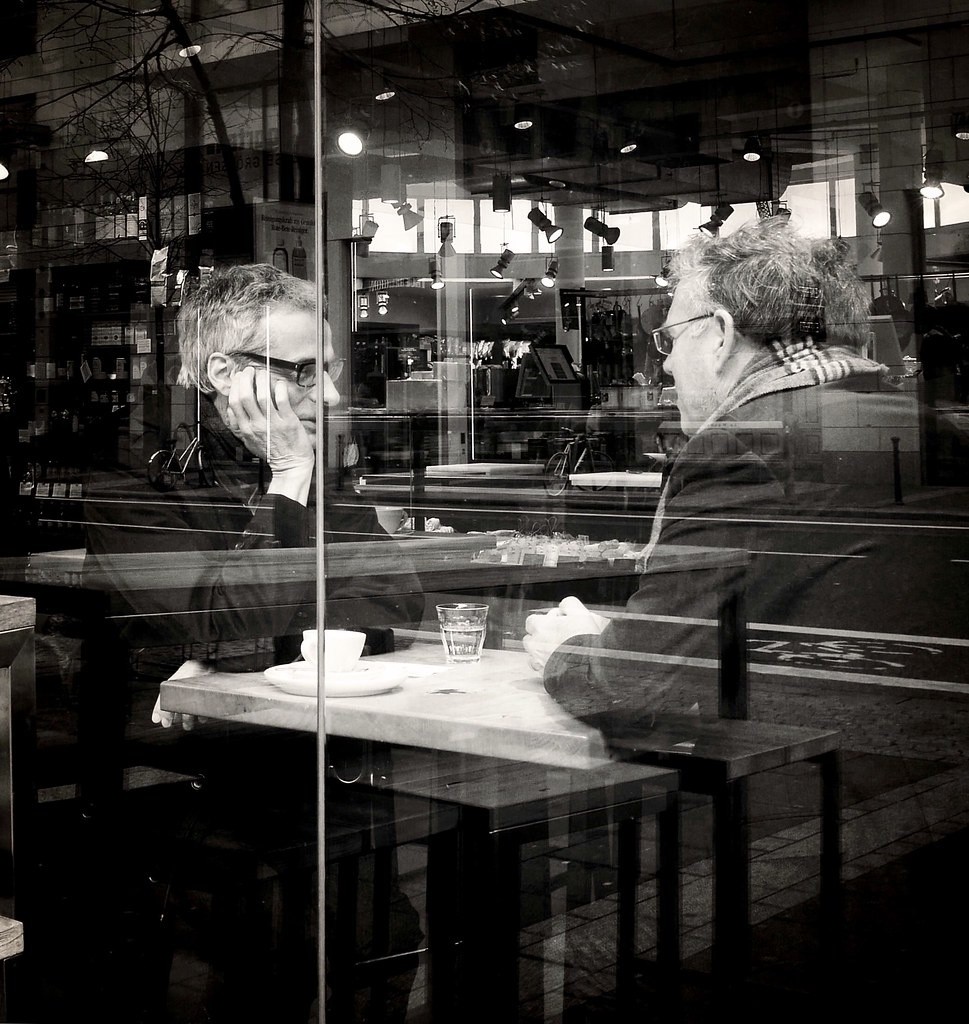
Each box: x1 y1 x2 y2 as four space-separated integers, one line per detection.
226 349 347 388
651 312 714 355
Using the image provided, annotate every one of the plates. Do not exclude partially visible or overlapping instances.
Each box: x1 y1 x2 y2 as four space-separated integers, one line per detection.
264 661 402 697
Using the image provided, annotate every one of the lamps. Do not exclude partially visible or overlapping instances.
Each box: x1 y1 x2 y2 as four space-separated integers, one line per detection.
490 243 516 279
381 162 402 204
362 220 379 244
437 178 457 258
663 114 704 169
376 290 390 316
584 120 639 272
332 86 395 158
654 250 672 286
428 178 446 290
358 294 370 318
858 39 893 229
497 253 559 326
741 136 791 230
492 99 564 243
698 96 734 240
951 112 969 140
919 26 946 201
397 203 423 231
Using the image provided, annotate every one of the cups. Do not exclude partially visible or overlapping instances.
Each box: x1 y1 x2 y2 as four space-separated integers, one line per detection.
436 603 489 665
375 507 408 534
300 630 367 670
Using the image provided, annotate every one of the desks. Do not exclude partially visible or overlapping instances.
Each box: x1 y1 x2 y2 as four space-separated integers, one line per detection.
570 470 663 510
0 526 751 1024
158 638 629 1024
425 462 545 485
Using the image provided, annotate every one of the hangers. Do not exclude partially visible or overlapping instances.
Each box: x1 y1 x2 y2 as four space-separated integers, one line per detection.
921 327 948 341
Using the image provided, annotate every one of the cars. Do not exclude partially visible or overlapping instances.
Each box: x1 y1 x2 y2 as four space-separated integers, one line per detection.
936 400 968 436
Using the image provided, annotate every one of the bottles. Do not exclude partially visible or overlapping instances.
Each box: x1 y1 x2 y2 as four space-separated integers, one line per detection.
33 191 139 247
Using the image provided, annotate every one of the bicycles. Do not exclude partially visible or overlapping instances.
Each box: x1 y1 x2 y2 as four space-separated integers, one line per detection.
148 423 216 489
543 425 615 498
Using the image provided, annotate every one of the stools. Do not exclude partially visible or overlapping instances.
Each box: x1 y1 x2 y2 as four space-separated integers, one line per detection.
321 740 682 1024
570 707 843 1024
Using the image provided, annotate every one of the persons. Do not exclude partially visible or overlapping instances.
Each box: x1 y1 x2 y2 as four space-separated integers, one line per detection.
523 221 969 862
13 263 424 1023
